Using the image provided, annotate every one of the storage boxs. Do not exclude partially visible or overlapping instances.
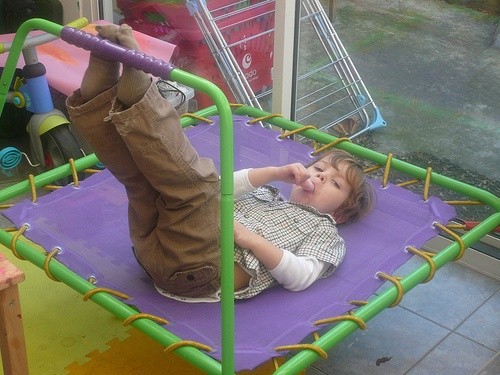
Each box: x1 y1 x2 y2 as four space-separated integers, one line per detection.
117 1 277 110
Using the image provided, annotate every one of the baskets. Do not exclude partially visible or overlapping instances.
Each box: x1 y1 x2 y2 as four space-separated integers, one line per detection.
116 0 276 111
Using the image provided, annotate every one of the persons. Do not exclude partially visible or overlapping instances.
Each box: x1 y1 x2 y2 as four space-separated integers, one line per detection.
62 23 370 302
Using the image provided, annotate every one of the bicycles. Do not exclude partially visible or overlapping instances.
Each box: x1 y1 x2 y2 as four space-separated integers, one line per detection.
0 17 92 183
